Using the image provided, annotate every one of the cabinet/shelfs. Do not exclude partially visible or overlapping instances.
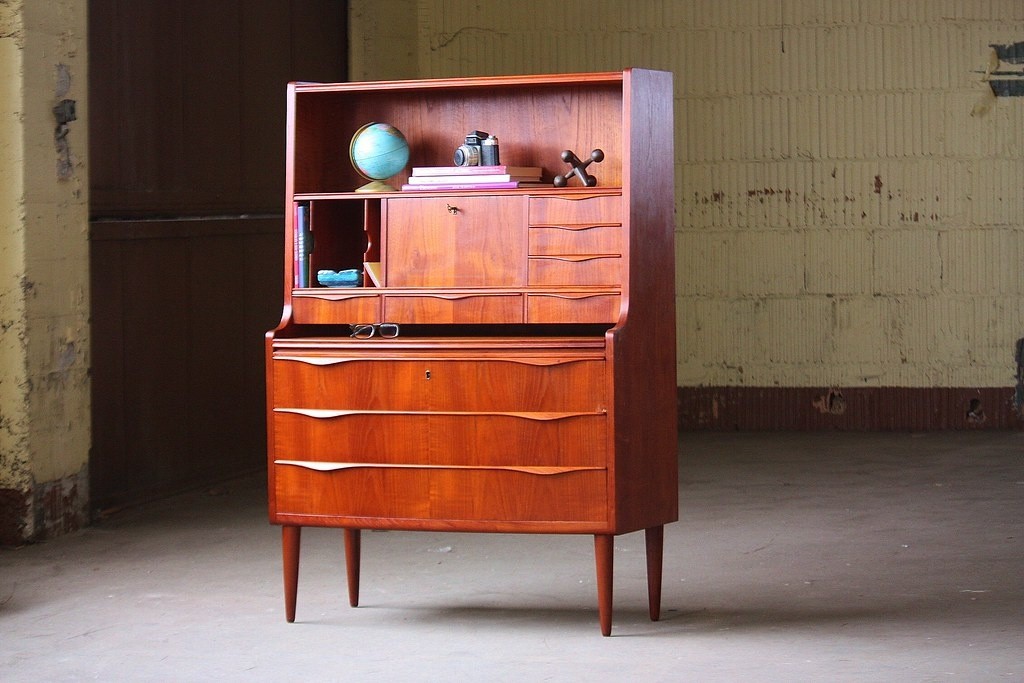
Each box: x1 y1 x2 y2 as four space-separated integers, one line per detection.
265 67 676 636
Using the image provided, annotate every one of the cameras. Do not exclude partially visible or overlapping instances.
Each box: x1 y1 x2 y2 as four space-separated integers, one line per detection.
453 130 500 167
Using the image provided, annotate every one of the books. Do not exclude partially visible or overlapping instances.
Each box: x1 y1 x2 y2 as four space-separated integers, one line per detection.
402 167 554 190
293 201 314 288
364 262 382 287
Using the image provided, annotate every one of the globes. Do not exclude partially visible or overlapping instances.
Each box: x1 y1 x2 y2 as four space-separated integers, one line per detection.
348 120 411 193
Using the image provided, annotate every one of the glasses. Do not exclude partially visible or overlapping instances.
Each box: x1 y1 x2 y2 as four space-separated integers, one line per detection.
348 324 399 340
